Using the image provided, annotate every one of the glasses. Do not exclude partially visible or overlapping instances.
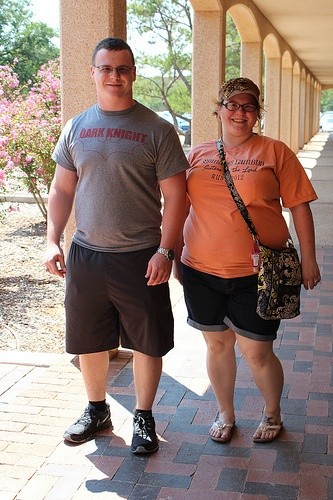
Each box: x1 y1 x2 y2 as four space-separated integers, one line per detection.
94 65 134 74
222 102 259 112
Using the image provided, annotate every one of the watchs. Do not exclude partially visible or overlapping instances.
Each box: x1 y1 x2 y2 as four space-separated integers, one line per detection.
156 247 175 261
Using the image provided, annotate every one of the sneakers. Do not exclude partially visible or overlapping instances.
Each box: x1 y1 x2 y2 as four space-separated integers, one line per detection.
63 403 112 442
130 413 160 453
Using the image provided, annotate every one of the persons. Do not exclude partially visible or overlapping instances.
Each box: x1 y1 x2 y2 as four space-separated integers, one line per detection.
171 76 321 444
42 36 191 455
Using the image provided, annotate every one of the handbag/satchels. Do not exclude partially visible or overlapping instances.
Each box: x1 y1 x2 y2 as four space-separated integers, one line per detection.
256 246 301 320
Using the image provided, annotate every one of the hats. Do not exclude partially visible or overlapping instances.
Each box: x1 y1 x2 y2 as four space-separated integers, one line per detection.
218 78 260 103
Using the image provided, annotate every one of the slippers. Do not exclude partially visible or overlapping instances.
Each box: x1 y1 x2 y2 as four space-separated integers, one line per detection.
210 420 235 442
253 421 284 443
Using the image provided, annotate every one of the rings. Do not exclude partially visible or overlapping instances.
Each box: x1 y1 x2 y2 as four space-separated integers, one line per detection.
315 280 318 283
